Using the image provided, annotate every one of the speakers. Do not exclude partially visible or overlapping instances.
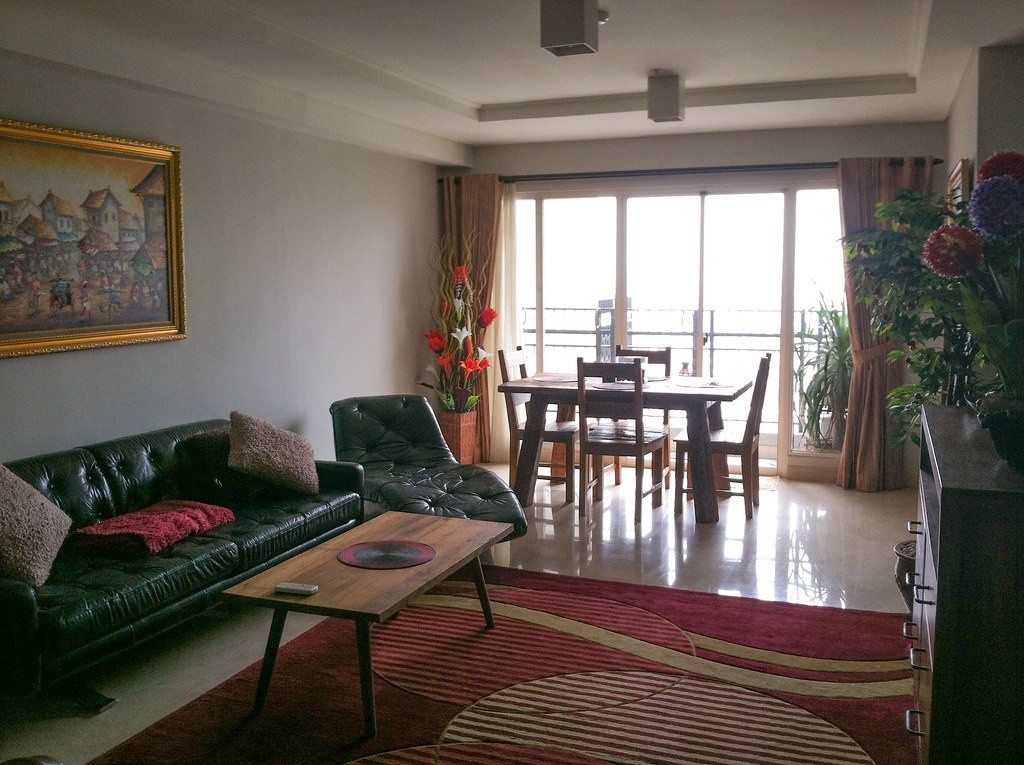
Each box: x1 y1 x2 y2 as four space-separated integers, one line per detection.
648 75 686 122
540 0 599 56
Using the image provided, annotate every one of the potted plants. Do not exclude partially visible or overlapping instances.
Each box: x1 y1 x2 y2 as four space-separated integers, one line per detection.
839 189 999 611
794 293 854 452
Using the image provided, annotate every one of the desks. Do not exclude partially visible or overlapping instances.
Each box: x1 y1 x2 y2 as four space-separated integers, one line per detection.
498 372 752 523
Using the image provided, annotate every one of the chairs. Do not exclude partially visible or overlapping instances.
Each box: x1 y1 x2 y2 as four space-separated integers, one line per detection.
329 395 527 543
498 344 772 523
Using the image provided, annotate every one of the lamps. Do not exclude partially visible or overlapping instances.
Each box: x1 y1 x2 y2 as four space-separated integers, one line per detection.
540 0 599 57
647 68 685 122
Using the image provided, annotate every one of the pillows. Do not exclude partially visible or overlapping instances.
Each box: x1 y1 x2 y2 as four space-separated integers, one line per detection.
188 411 319 504
0 464 72 588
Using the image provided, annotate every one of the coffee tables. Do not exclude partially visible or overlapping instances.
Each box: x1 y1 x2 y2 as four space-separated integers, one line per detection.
222 511 515 738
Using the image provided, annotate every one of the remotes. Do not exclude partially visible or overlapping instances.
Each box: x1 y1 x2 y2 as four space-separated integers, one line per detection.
276 582 318 595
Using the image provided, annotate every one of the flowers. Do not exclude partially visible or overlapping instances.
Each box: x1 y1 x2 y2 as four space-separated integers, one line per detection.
923 152 1023 404
416 225 498 412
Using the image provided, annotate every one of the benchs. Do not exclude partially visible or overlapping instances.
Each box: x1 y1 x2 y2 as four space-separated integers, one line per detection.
0 419 365 712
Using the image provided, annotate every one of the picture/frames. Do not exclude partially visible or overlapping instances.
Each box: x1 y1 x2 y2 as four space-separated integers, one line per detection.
0 118 187 359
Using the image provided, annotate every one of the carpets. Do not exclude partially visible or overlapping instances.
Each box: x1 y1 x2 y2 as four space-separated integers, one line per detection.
86 561 918 765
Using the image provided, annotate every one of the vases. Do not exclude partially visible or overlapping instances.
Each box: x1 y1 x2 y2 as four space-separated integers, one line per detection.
976 398 1024 472
434 412 477 463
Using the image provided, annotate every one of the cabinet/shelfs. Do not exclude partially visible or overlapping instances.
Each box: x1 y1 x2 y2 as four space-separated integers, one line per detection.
903 405 1024 765
